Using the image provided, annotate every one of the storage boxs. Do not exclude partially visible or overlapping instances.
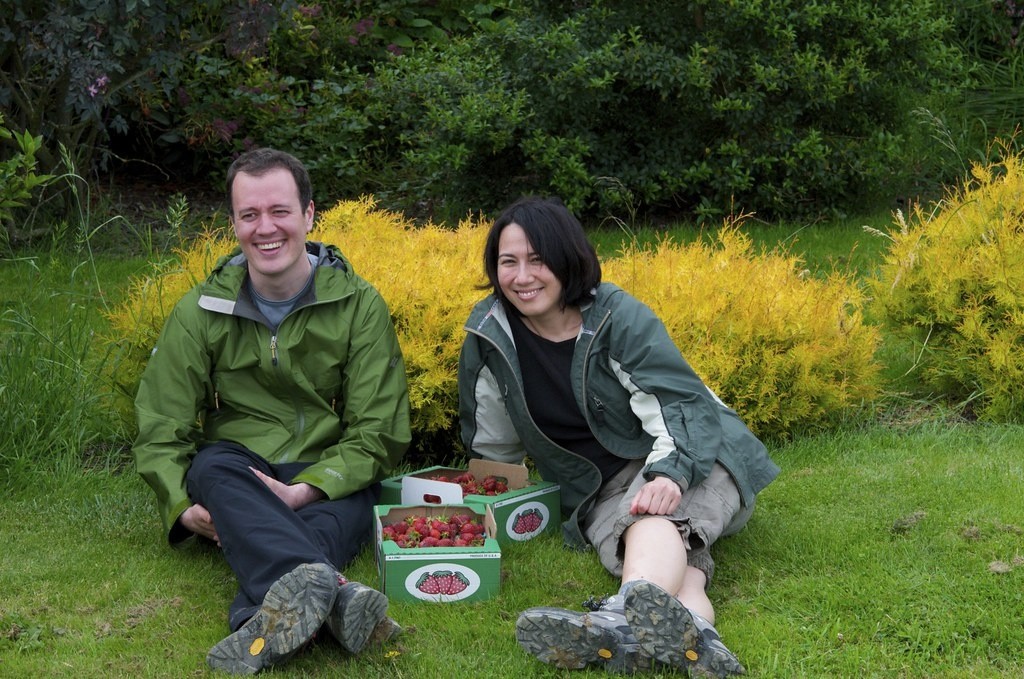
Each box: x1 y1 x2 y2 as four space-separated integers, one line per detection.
371 502 502 604
381 459 560 555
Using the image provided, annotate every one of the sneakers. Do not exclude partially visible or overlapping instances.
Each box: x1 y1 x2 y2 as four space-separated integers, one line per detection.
515 594 669 674
624 582 740 679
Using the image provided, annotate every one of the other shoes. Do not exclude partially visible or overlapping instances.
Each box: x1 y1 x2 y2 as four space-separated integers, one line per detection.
206 563 336 674
326 571 403 656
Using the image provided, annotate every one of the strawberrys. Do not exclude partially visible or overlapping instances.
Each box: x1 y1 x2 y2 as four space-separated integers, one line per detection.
382 512 485 549
419 472 508 505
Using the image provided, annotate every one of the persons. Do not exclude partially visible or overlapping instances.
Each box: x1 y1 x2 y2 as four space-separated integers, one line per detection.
131 146 412 677
458 196 781 679
